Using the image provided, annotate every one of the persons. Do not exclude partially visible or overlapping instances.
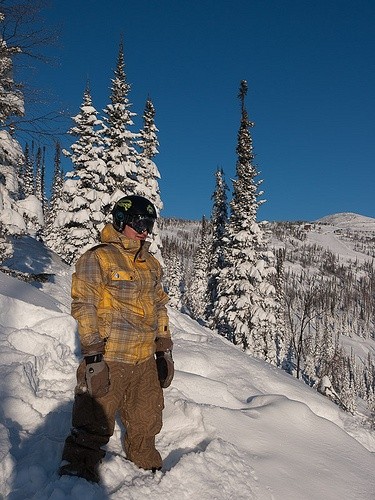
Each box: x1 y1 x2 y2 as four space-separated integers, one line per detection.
58 195 174 482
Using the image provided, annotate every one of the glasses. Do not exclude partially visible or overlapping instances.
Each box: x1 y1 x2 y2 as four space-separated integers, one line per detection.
127 215 154 234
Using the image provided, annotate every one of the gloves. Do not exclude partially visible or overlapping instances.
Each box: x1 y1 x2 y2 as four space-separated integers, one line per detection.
82 343 112 398
154 337 174 388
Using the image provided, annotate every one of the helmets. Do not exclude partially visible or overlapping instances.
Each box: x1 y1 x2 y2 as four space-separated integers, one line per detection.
111 196 157 233
